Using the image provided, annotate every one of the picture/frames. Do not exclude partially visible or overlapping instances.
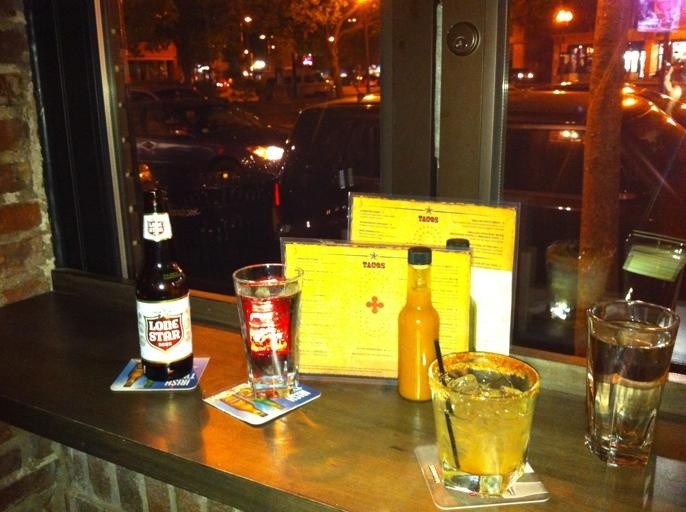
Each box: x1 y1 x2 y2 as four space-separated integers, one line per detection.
279 236 475 387
347 191 522 356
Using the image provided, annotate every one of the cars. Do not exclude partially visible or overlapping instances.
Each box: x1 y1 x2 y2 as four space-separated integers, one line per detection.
138 64 332 135
507 65 686 126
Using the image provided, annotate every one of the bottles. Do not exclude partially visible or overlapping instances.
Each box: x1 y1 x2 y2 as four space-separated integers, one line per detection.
124 361 159 388
396 247 440 402
135 186 195 382
446 237 476 351
222 387 284 417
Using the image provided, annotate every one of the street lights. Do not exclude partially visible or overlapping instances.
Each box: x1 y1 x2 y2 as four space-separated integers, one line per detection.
237 14 253 69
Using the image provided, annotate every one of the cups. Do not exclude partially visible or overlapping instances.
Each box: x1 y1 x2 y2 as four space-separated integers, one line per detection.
427 351 542 497
583 300 680 467
232 263 305 398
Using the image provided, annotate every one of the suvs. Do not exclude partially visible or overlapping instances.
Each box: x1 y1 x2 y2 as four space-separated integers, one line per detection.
126 81 285 278
270 92 686 346
